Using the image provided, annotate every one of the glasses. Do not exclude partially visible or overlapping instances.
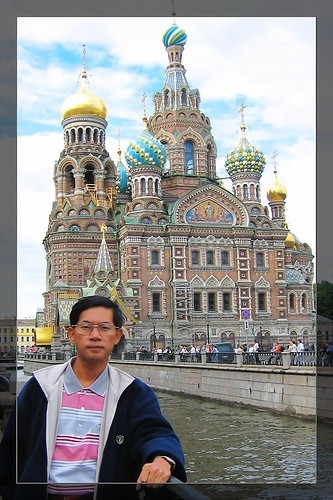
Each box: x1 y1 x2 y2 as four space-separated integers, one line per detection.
72 323 119 335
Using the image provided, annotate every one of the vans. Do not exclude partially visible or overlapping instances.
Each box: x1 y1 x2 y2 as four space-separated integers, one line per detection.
198 343 235 364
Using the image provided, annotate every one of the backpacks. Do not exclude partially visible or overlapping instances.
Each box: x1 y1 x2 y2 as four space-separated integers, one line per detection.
281 346 285 352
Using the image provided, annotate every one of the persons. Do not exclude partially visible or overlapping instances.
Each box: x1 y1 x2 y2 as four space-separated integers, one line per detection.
1 295 187 499
140 344 188 362
189 338 306 365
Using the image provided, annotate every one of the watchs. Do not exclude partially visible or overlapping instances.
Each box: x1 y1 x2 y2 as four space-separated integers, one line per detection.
156 455 176 472
